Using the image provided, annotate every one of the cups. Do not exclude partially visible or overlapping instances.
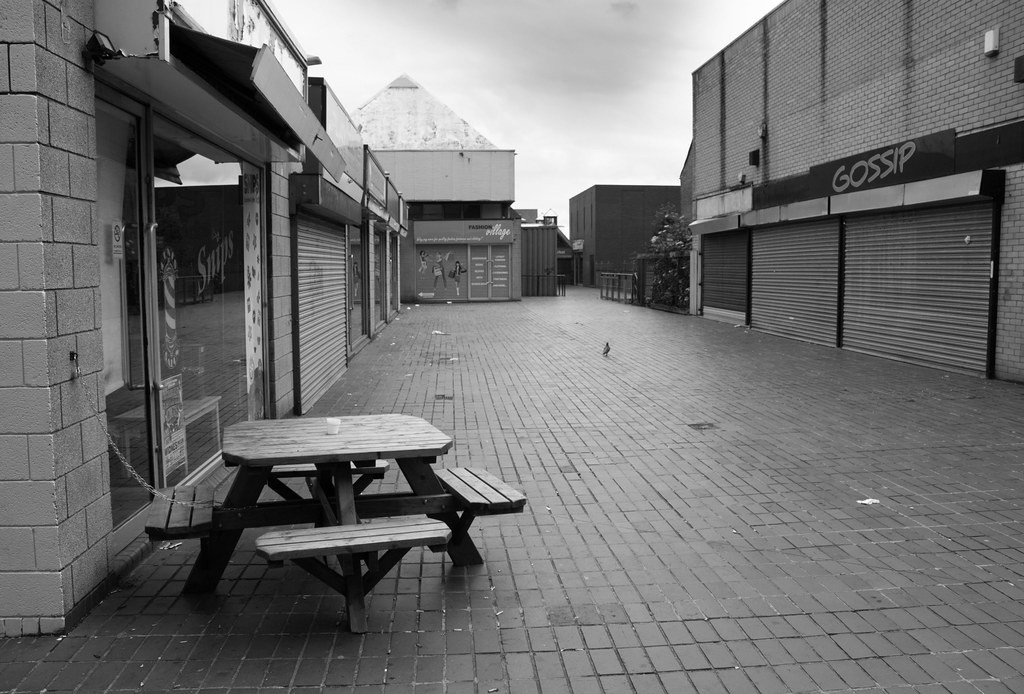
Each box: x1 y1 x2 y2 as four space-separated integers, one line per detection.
327 418 340 434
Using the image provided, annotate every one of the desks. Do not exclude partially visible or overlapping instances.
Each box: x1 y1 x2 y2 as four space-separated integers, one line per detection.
179 413 485 636
114 396 222 481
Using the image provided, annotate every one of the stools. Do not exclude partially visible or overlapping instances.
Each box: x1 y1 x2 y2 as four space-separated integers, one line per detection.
251 517 453 562
268 459 391 477
143 483 215 540
435 465 529 517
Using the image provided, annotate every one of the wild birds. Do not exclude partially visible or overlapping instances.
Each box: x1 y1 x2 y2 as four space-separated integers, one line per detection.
603 342 610 356
